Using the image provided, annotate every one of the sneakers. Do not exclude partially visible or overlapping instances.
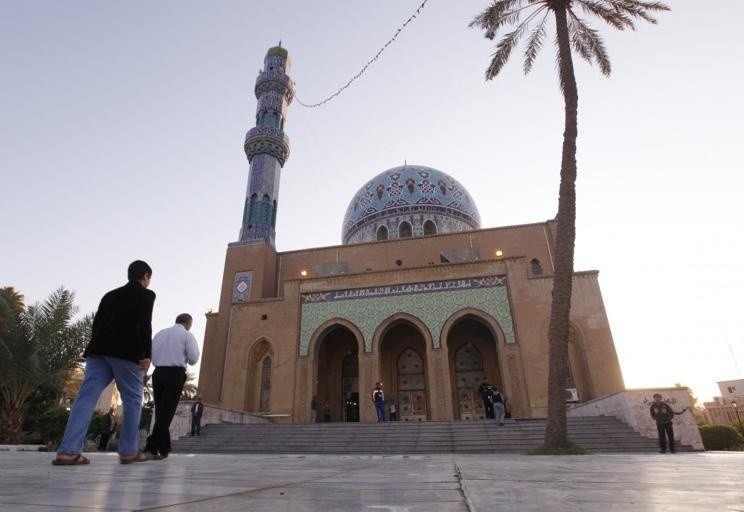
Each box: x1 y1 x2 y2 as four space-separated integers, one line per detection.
144 446 168 457
660 448 675 454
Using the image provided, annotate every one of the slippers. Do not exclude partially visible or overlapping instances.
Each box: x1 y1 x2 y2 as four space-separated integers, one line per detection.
120 451 154 463
52 454 90 464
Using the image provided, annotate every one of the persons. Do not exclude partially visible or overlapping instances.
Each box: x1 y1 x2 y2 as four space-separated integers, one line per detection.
372 383 387 423
490 386 505 427
98 407 116 451
51 260 156 466
191 397 203 436
311 396 318 423
479 377 495 419
649 394 676 454
323 400 331 423
389 400 398 421
144 313 200 457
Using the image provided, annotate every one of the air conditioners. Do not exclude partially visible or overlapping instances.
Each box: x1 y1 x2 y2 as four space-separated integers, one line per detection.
564 389 579 402
